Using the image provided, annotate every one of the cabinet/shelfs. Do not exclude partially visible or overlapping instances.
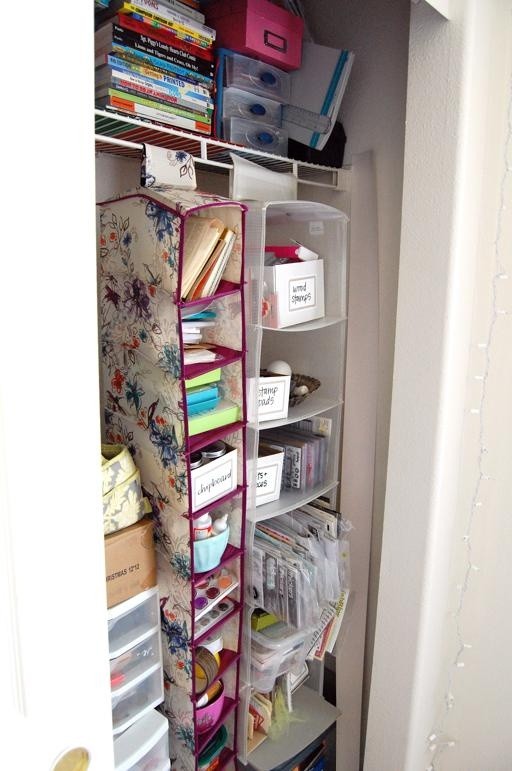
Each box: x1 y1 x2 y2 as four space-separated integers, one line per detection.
241 195 350 769
102 188 241 770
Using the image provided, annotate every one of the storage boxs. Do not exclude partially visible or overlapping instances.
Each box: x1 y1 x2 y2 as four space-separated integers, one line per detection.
103 665 166 736
255 449 284 508
219 54 294 105
221 87 282 127
126 730 168 771
223 117 288 162
102 629 163 695
200 1 302 71
102 520 156 609
260 370 290 421
261 247 326 329
105 704 168 770
187 449 238 515
102 586 163 659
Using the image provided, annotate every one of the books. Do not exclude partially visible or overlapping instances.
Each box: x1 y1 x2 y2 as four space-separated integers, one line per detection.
178 301 224 418
182 215 236 301
93 0 217 133
